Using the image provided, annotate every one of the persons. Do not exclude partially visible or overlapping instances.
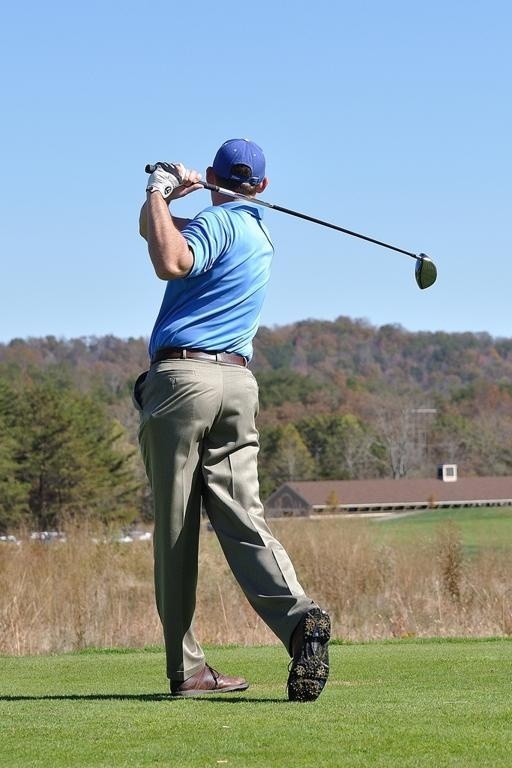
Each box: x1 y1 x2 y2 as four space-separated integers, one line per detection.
132 137 330 703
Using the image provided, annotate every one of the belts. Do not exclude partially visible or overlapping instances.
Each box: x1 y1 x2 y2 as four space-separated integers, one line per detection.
151 347 249 367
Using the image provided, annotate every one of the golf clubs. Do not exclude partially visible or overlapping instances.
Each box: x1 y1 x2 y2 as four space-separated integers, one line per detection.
146 164 438 290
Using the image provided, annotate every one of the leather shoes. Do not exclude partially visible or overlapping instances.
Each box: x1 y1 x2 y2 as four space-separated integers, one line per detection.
169 661 249 696
287 607 332 703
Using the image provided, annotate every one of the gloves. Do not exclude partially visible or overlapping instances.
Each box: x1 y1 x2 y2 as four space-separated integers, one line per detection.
146 161 186 198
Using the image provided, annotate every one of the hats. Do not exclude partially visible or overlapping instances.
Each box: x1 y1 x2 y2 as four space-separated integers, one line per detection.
213 138 266 186
